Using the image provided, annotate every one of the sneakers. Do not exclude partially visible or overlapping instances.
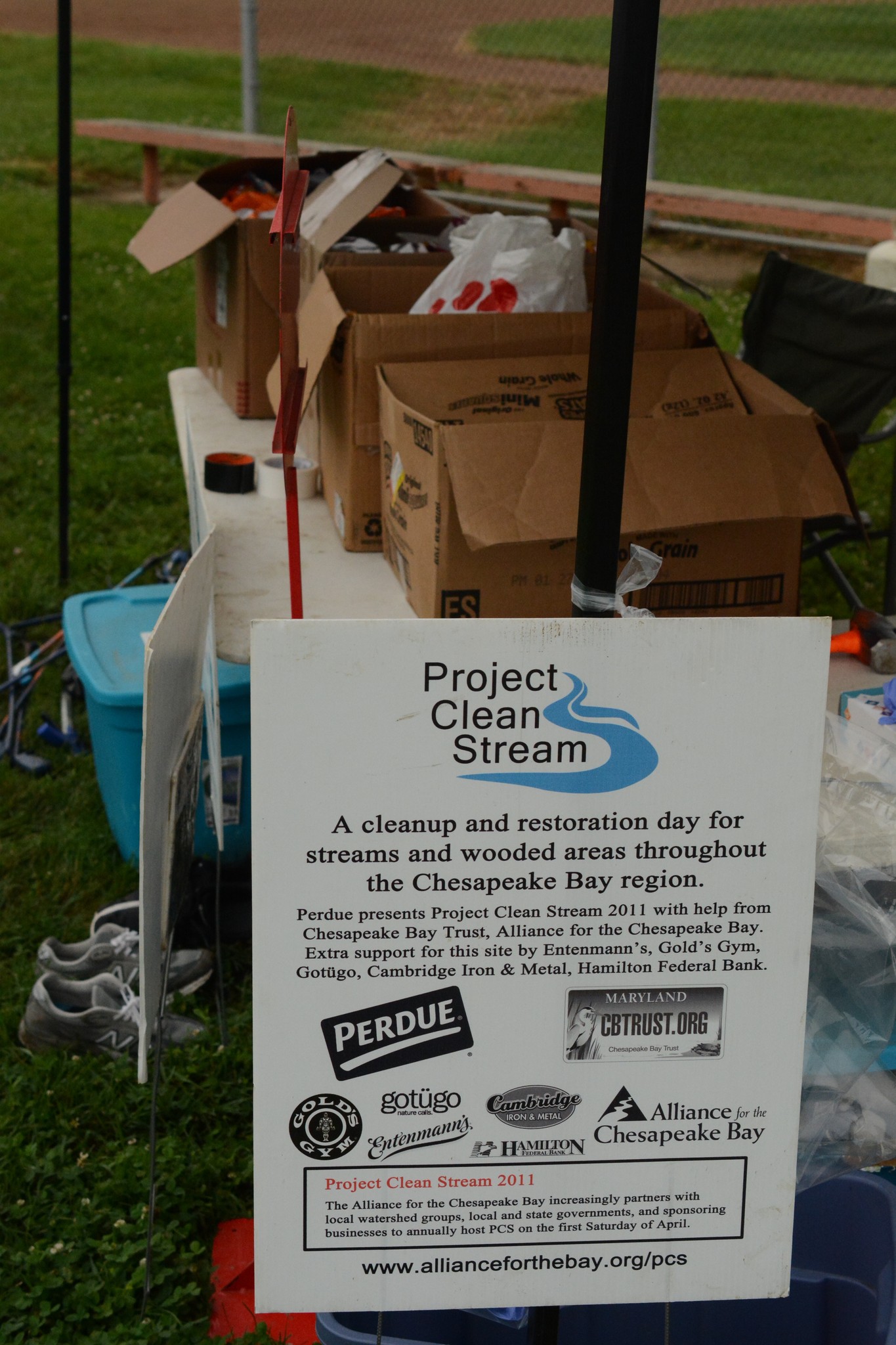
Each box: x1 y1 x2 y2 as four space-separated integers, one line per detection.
36 922 216 1005
18 973 206 1054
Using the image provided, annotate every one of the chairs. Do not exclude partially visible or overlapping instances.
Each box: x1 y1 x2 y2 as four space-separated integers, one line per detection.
740 248 896 616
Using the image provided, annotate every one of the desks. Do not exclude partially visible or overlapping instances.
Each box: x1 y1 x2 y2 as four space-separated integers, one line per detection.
170 364 419 664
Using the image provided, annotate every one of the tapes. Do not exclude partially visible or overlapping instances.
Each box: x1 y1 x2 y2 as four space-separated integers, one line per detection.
203 452 255 493
259 456 320 503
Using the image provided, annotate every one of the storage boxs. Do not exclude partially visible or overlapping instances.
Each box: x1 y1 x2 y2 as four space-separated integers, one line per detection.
121 145 874 617
59 585 250 868
315 1169 896 1345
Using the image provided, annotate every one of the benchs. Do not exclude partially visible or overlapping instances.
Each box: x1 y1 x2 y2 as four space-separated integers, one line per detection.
73 116 896 243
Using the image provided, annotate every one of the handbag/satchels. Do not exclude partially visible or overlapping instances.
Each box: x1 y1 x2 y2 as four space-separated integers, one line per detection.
408 211 589 314
